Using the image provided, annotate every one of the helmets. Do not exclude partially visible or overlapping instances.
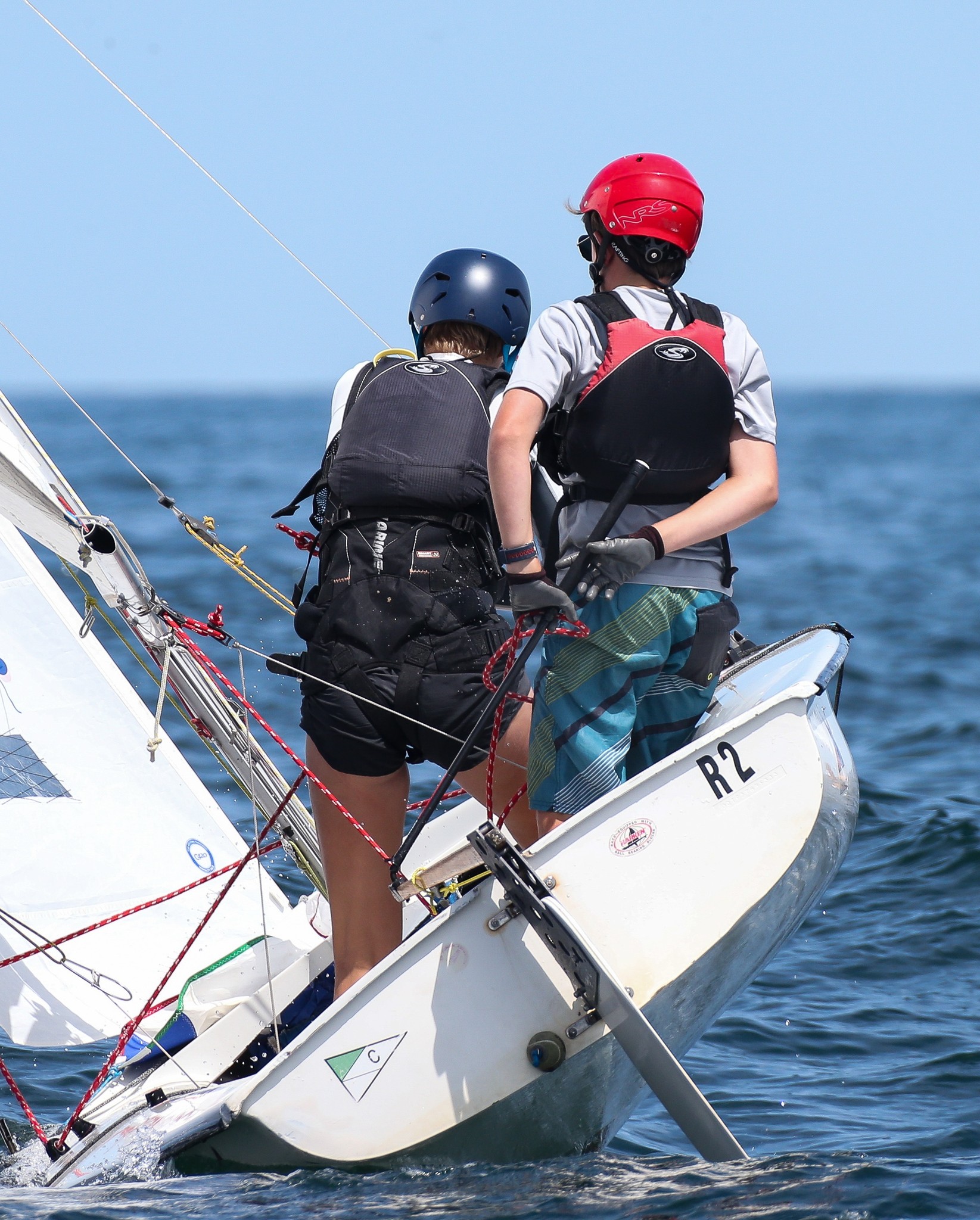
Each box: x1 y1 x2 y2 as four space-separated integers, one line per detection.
579 152 705 258
408 248 531 347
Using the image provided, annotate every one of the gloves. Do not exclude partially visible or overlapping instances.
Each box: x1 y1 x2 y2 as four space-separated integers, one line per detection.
554 525 665 600
505 568 578 632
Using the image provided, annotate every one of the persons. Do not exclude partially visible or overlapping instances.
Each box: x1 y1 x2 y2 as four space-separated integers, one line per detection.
484 152 776 837
297 246 549 1008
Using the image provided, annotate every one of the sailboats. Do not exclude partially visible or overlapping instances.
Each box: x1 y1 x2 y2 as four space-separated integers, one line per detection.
1 0 861 1194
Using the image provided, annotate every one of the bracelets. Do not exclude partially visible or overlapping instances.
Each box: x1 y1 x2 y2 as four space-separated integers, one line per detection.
498 541 540 564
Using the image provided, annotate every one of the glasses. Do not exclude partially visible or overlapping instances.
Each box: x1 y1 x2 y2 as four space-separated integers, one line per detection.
576 230 597 262
